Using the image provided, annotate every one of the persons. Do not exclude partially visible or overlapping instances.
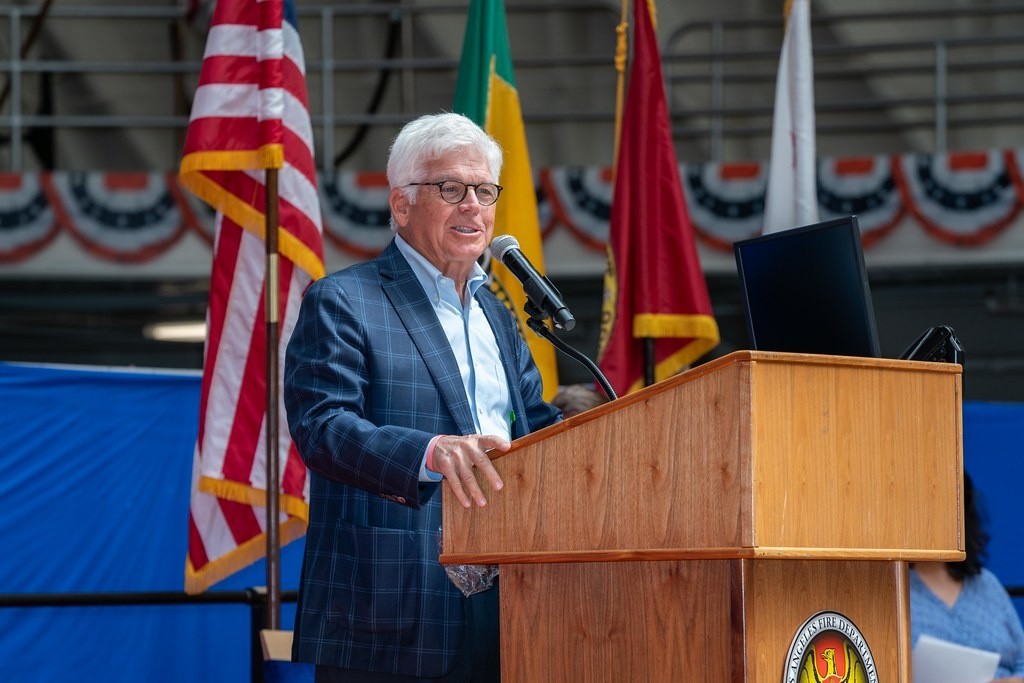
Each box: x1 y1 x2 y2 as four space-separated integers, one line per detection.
548 384 607 421
906 471 1024 683
276 110 559 683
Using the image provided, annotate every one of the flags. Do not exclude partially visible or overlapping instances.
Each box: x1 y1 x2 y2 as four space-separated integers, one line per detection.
593 0 722 404
172 0 324 593
456 1 559 415
761 0 820 236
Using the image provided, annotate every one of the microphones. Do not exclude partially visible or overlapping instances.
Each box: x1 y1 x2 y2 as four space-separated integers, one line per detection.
491 234 576 332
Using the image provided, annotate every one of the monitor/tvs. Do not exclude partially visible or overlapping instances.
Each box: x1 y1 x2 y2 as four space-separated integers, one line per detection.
732 214 882 360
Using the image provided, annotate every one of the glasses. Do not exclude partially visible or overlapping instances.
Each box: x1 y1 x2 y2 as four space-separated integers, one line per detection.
401 180 503 206
